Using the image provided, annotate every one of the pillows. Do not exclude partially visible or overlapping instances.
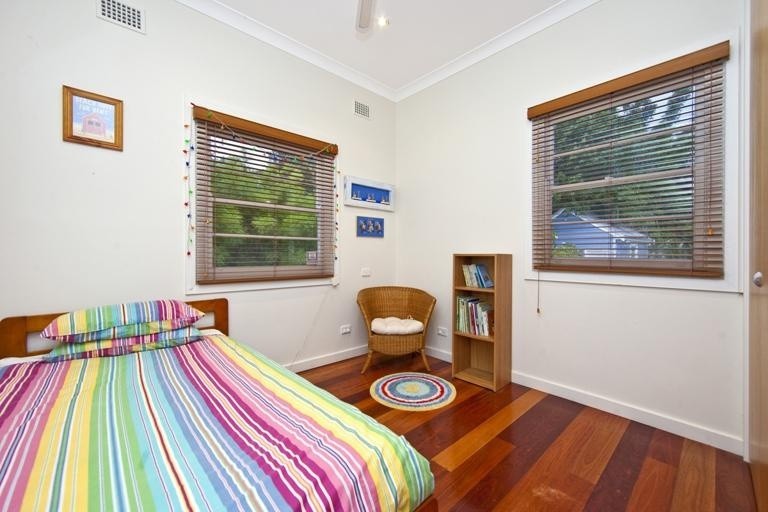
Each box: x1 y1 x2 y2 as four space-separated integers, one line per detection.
39 299 204 342
39 324 201 362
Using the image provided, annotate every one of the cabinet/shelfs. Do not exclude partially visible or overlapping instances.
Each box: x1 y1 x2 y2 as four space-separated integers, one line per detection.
451 254 512 392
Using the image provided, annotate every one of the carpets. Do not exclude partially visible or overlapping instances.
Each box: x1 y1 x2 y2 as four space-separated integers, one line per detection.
368 370 456 412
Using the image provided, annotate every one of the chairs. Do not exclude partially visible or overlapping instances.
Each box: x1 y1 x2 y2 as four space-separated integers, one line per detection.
357 286 437 374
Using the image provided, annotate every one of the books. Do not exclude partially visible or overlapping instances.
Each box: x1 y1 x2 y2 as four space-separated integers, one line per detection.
456 295 494 339
461 263 494 289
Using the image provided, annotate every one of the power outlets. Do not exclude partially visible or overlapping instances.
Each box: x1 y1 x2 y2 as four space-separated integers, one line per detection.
437 326 448 337
341 324 351 334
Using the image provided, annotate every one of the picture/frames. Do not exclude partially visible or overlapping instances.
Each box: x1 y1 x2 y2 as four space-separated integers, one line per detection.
343 175 395 211
61 85 124 151
353 213 388 244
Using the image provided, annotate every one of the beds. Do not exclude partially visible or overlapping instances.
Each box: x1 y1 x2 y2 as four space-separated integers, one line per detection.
0 297 436 511
